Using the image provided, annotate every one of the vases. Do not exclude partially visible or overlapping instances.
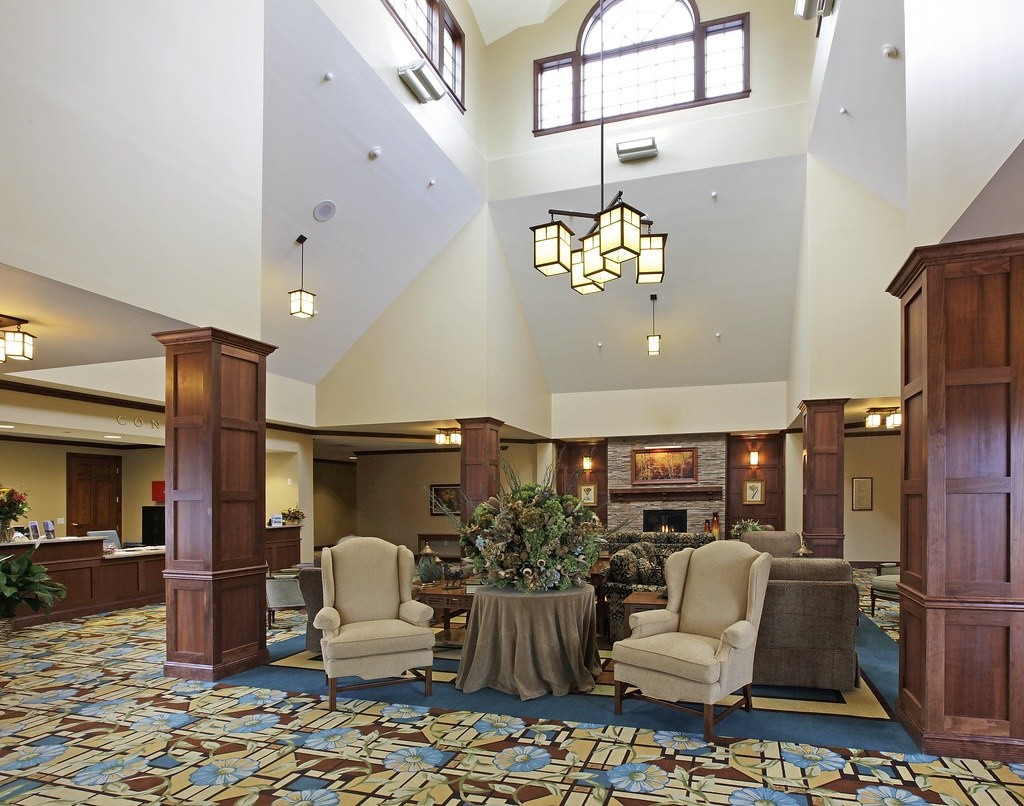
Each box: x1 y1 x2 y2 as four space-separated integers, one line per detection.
0 519 12 543
286 520 299 525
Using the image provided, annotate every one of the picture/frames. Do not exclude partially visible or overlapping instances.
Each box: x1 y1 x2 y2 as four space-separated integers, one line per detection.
430 483 461 516
577 482 599 507
631 446 698 484
742 479 766 505
851 477 873 512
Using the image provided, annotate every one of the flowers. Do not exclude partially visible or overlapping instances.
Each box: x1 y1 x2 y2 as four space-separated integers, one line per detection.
0 479 32 522
421 441 644 595
730 519 762 540
280 503 306 524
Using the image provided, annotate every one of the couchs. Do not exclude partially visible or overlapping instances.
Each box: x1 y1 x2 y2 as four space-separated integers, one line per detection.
752 557 862 692
298 567 323 653
605 532 717 545
740 531 801 557
600 542 668 644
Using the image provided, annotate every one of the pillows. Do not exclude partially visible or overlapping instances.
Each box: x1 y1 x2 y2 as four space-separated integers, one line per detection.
638 558 665 586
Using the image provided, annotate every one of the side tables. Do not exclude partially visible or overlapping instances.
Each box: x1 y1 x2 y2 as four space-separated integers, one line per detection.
621 591 668 639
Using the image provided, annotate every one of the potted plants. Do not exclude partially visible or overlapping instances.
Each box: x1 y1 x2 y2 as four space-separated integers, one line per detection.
0 533 67 643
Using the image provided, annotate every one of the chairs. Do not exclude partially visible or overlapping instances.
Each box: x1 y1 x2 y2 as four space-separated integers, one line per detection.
266 560 306 630
612 540 774 743
87 530 122 549
870 563 900 618
313 536 435 712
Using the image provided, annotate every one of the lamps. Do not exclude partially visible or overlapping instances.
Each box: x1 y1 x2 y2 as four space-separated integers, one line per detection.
582 455 593 470
793 0 834 21
864 407 902 430
287 234 316 319
0 314 37 365
398 59 448 104
749 451 759 466
435 428 461 446
646 293 662 356
529 0 669 296
615 135 659 163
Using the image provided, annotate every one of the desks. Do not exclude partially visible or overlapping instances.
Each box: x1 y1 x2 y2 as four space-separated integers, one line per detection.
589 562 608 636
418 586 475 649
416 533 466 562
454 583 603 701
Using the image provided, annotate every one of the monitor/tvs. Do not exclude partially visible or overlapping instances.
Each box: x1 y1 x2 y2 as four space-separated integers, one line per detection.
141 506 165 546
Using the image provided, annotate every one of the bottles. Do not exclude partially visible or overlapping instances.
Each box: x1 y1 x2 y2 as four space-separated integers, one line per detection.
712 511 721 541
704 519 711 533
